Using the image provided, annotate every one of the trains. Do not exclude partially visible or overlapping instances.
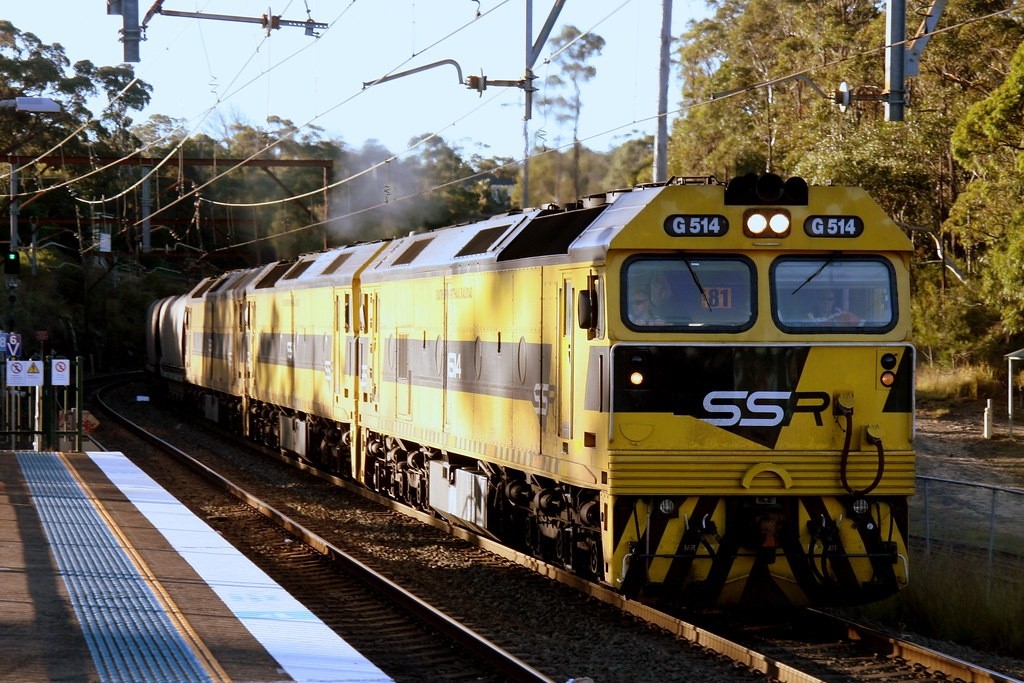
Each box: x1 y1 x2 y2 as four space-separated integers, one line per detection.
145 173 919 609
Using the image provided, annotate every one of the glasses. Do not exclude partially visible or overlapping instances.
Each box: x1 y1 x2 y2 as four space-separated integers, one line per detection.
819 296 833 301
630 299 649 305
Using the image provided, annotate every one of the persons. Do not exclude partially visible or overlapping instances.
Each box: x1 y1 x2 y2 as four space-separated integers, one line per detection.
801 288 840 321
627 291 663 327
